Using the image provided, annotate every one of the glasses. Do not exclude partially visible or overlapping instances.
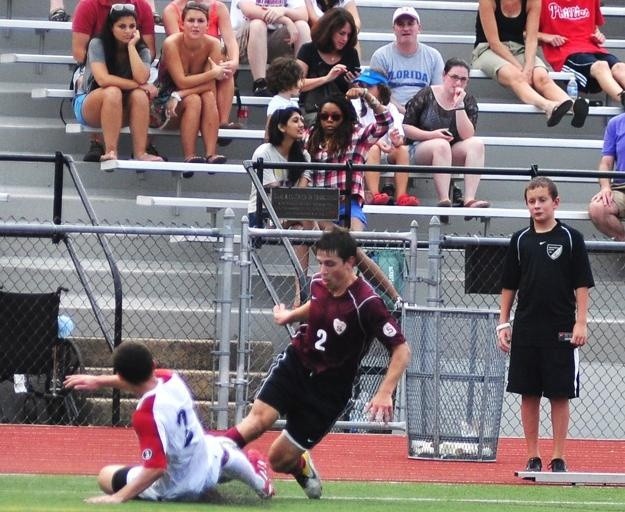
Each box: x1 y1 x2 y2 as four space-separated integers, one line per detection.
108 2 137 17
183 0 210 15
446 73 471 83
317 110 343 122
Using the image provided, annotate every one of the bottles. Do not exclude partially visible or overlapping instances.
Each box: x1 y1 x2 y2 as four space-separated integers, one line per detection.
238 104 249 129
566 76 577 102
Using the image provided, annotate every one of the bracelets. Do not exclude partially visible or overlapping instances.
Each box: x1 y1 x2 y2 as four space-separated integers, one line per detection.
495 323 512 329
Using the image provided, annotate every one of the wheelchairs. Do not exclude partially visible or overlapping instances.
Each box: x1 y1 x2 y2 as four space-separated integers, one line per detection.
0 281 87 424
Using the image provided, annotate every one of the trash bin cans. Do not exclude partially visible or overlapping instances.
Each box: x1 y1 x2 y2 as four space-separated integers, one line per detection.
400 301 510 462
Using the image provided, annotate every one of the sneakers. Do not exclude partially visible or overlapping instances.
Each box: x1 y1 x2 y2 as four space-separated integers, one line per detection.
152 12 164 27
549 457 570 472
82 135 106 164
46 7 71 22
289 446 326 501
252 78 273 97
522 455 545 482
245 445 277 500
130 140 169 163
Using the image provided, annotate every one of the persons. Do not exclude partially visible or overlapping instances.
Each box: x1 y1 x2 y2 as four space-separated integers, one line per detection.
471 1 625 127
493 176 596 479
50 1 360 176
247 5 490 308
213 231 410 499
589 90 624 243
63 340 274 506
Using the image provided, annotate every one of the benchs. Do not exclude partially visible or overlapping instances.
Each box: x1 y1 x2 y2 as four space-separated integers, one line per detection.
0 1 625 345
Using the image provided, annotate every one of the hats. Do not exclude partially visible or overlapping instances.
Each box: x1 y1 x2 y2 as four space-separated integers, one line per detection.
352 67 390 87
391 5 422 27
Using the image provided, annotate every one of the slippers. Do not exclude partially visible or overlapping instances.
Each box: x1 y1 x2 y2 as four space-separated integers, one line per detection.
546 98 574 128
569 94 592 130
435 198 456 225
463 197 491 222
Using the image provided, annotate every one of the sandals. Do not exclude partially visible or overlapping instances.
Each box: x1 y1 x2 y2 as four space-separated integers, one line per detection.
206 153 228 177
370 191 392 205
180 154 207 180
394 192 421 207
